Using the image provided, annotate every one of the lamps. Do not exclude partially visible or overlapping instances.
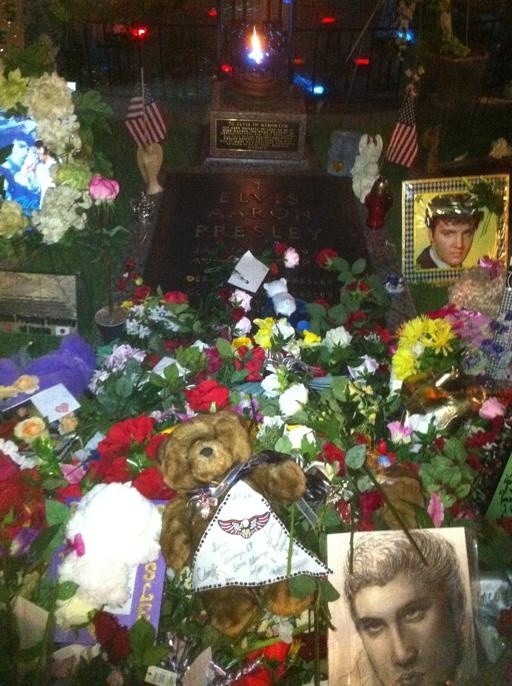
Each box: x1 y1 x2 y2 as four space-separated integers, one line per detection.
246 28 272 72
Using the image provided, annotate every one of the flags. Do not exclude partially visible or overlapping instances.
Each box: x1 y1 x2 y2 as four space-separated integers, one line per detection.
123 80 168 150
386 89 419 170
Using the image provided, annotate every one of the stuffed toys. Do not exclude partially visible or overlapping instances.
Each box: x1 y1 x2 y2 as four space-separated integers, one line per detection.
157 410 318 638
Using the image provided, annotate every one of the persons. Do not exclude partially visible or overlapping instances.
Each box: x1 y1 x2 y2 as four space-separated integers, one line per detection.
415 193 482 269
337 532 477 686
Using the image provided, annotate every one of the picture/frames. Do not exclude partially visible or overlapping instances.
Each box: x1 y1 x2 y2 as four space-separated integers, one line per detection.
400 173 512 287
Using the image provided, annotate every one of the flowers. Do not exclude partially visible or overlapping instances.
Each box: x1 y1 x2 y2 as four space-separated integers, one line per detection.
2 33 123 269
1 250 511 686
